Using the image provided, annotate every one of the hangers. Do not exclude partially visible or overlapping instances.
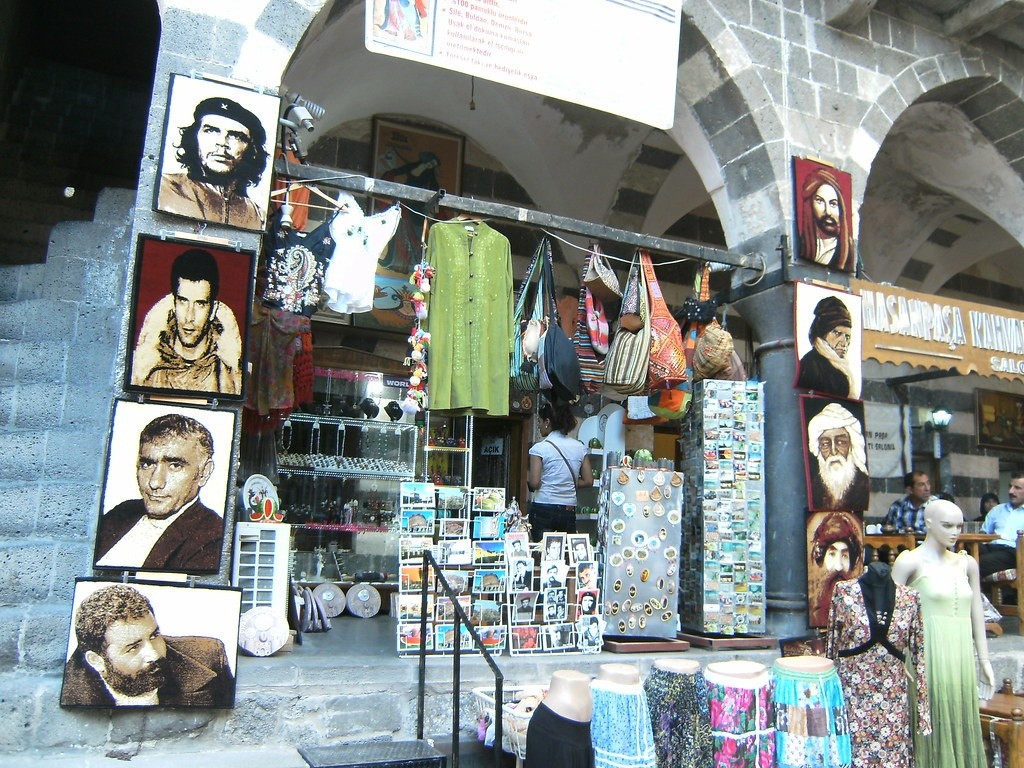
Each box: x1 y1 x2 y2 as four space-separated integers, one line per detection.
270 182 349 212
456 213 482 224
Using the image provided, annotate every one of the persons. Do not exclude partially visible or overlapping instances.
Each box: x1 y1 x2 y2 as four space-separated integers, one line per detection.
890 500 994 768
579 591 595 615
527 657 851 768
397 489 538 649
581 618 601 650
574 541 594 590
807 403 869 508
526 402 594 609
135 247 237 396
825 562 932 768
973 471 1024 589
878 470 965 565
60 586 233 707
542 565 568 647
800 166 856 272
814 514 865 626
800 297 857 396
96 414 224 569
544 539 561 561
157 97 265 227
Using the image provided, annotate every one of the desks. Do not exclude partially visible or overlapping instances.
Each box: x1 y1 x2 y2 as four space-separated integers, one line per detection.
866 532 1001 565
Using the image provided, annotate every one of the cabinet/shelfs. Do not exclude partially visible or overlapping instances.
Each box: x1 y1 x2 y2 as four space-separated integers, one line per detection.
274 411 418 590
577 402 627 548
422 409 473 528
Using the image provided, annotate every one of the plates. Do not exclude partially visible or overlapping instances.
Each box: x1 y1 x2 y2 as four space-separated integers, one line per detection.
243 474 279 511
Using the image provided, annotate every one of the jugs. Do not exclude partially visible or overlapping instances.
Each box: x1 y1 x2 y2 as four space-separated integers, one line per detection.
880 521 896 534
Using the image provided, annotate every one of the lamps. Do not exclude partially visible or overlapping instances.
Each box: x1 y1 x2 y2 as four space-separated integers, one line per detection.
924 406 953 432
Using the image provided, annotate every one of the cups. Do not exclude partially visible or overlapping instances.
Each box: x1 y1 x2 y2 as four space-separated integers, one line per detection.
874 524 883 534
866 524 877 534
963 521 980 534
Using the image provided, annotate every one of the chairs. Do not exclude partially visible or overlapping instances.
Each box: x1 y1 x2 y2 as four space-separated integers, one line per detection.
986 530 1024 636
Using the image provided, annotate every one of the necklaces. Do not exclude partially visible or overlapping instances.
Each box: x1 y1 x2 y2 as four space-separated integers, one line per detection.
278 371 402 463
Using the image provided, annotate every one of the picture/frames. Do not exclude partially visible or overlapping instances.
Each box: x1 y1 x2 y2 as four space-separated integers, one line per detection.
972 386 1024 452
350 114 467 331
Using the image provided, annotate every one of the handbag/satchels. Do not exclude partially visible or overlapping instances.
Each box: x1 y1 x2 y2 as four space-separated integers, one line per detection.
507 235 735 420
